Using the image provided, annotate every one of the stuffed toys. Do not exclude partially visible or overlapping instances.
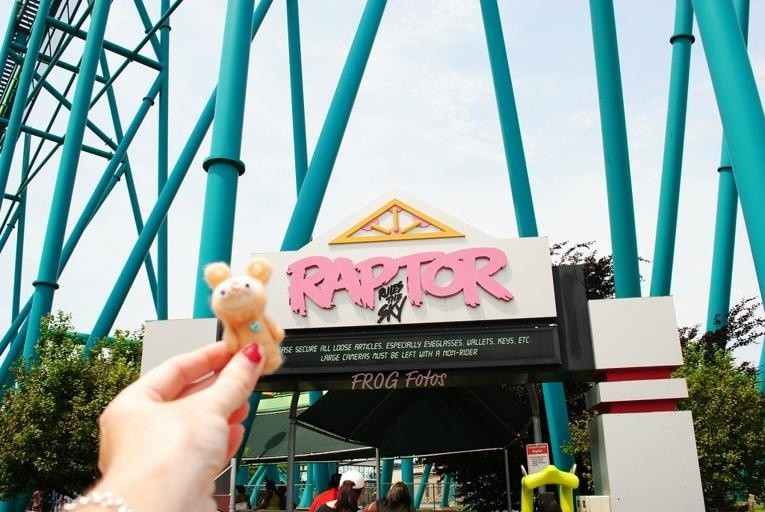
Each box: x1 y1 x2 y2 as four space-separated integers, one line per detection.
203 261 286 375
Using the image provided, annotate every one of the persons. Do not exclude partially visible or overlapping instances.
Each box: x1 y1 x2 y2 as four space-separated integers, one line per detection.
234 477 296 511
52 342 266 512
30 487 70 512
308 465 417 512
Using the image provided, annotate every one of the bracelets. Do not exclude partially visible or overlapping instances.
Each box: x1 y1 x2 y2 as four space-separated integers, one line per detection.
62 492 136 512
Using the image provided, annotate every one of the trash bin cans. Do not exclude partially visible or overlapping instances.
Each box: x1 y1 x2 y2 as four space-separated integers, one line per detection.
576 495 610 512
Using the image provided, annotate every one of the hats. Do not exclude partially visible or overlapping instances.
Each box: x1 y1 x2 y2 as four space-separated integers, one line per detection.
340 470 364 489
